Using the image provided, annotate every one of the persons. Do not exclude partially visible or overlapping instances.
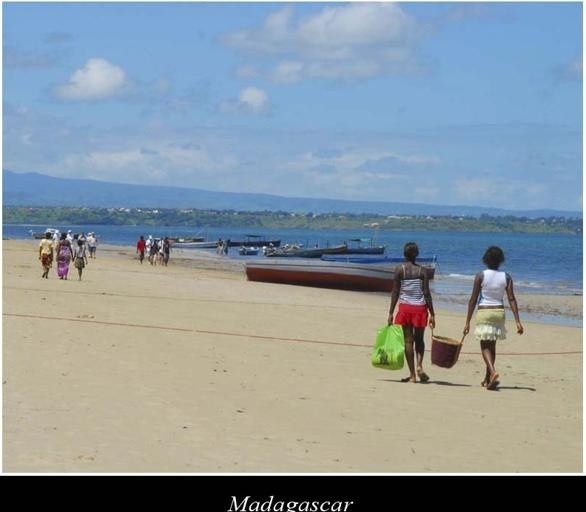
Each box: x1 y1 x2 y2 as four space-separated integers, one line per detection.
39 229 98 281
387 241 436 383
136 234 172 267
216 236 230 255
463 245 524 390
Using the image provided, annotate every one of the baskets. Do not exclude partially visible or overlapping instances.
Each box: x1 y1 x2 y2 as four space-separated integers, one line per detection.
431 327 466 368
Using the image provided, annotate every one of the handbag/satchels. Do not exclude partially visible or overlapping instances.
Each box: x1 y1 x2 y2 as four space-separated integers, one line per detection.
372 324 405 370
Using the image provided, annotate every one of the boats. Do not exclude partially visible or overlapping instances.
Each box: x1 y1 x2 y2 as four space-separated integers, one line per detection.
34 230 78 240
263 243 346 257
172 241 223 249
348 237 374 242
154 237 205 245
245 258 396 293
347 245 387 255
321 254 438 280
228 235 282 246
238 247 259 255
